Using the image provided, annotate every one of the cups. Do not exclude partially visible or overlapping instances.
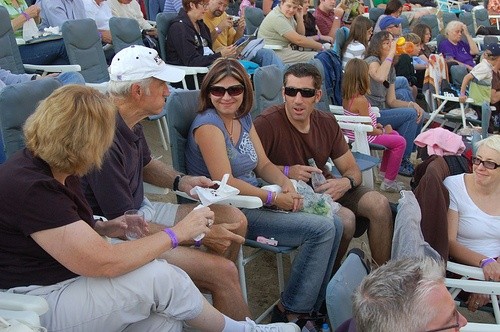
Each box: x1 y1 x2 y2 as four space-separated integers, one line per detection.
124 209 145 240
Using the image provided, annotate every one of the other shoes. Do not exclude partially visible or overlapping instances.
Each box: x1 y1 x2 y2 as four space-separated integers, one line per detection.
399 156 415 176
271 305 306 330
379 181 407 192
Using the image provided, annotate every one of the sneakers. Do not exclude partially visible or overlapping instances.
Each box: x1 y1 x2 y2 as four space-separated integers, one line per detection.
235 317 301 332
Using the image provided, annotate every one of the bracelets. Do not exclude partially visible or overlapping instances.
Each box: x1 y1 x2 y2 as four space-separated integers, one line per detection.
215 27 221 33
163 228 178 248
195 241 201 247
385 57 393 62
408 101 412 108
461 93 465 95
335 16 338 20
284 166 289 176
266 191 277 206
317 34 320 40
173 174 184 191
22 12 30 21
480 258 496 268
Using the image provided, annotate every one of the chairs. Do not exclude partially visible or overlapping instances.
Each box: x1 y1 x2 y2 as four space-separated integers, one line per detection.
0 0 500 332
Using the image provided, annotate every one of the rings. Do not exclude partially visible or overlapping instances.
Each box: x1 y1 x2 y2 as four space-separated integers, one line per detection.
474 302 479 304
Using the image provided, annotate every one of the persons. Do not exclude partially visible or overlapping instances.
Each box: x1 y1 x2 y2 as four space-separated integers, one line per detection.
0 0 500 134
252 63 393 270
0 84 301 332
185 57 344 329
443 134 500 312
341 58 407 192
364 31 424 177
80 45 253 322
353 257 467 332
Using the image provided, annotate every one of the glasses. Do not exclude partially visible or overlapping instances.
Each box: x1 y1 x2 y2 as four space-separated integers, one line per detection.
194 2 209 8
425 303 460 332
210 84 245 97
387 24 399 28
472 157 500 170
284 86 317 98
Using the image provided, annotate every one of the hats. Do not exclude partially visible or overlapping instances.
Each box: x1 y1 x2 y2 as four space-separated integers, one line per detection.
109 44 186 83
485 42 500 56
379 15 402 30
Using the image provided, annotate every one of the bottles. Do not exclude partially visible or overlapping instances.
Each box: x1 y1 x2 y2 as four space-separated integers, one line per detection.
308 157 327 189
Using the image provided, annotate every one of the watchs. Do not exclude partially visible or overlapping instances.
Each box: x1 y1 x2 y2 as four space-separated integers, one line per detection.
344 175 356 190
